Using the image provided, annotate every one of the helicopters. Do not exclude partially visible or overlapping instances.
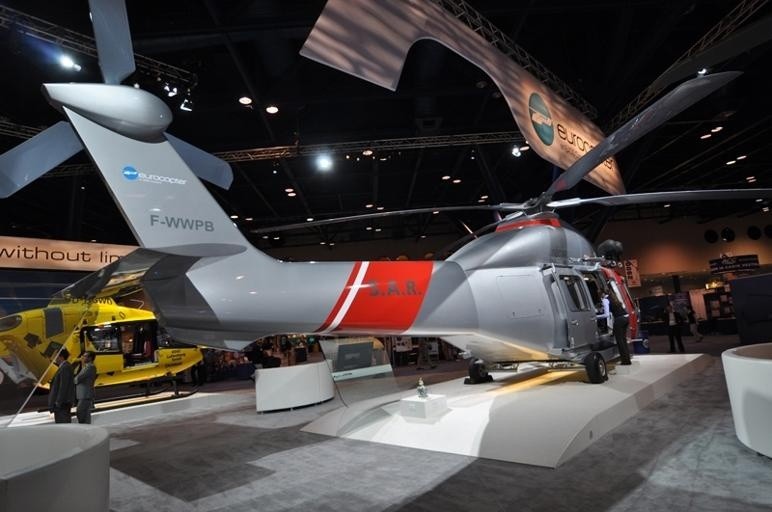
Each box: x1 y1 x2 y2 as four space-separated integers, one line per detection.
0 295 203 415
0 0 746 382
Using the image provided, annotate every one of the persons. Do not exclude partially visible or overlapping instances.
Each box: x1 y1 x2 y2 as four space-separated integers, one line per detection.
416 337 438 370
49 348 75 422
661 305 686 354
682 304 705 343
597 288 632 364
74 350 98 423
170 330 322 382
